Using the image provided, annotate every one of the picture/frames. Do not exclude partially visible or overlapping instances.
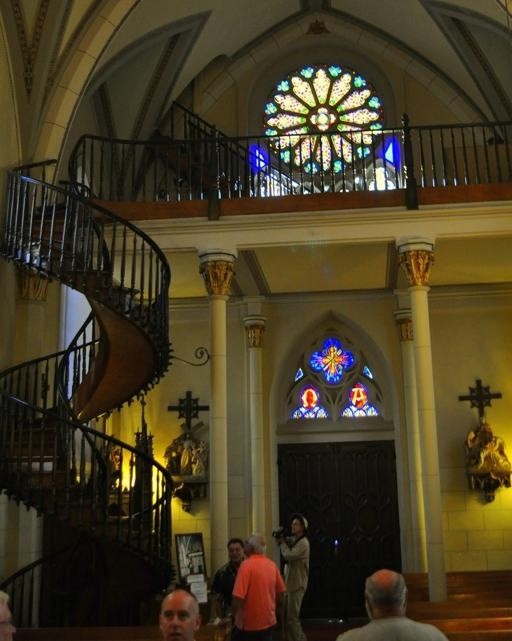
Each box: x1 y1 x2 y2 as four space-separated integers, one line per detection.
175 532 207 584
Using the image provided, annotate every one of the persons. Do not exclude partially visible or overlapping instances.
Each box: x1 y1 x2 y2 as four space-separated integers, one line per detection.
0 591 17 641
229 534 287 641
274 512 311 641
158 588 201 640
210 538 245 628
335 568 448 641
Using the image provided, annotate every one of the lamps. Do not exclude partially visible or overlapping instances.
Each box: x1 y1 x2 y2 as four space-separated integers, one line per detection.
262 63 385 177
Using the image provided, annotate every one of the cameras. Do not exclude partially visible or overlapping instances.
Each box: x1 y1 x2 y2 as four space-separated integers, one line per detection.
272 528 286 540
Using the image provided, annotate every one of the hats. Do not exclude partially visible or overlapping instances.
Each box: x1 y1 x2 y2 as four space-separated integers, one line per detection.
289 511 309 532
